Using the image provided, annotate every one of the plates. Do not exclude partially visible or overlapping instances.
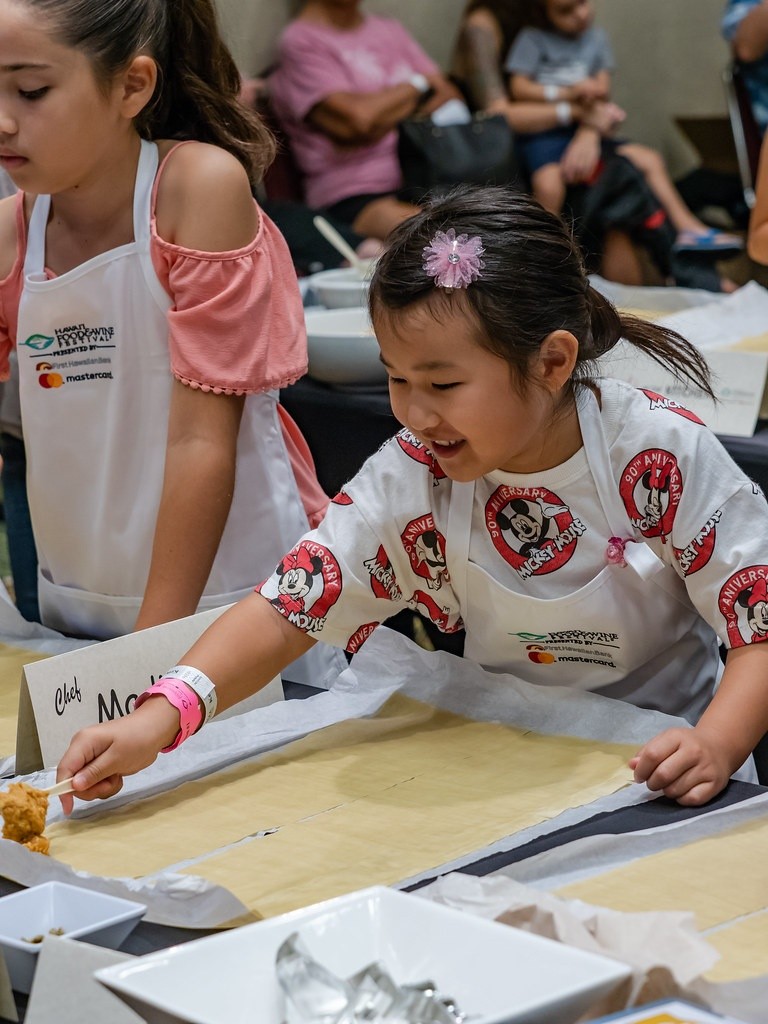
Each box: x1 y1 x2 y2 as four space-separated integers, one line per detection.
92 883 634 1024
592 997 742 1024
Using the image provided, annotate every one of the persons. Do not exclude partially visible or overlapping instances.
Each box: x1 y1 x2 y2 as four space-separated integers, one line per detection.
0 0 413 706
57 183 768 819
264 0 768 284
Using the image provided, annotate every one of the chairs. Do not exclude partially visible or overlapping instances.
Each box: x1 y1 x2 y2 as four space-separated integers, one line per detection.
253 55 768 289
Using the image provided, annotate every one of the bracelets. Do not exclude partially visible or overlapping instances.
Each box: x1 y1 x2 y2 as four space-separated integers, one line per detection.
545 85 557 101
556 102 572 126
161 665 217 734
410 75 430 92
134 679 202 754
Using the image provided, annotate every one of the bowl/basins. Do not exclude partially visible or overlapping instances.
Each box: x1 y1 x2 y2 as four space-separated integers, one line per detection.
309 258 384 309
0 881 147 995
303 308 391 391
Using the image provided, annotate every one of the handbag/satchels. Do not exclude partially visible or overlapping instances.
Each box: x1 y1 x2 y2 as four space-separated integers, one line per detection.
394 71 512 204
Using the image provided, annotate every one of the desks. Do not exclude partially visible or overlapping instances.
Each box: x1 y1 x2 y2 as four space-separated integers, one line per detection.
280 291 768 484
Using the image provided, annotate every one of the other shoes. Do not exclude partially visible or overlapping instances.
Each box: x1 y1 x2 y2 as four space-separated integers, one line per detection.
672 227 745 255
